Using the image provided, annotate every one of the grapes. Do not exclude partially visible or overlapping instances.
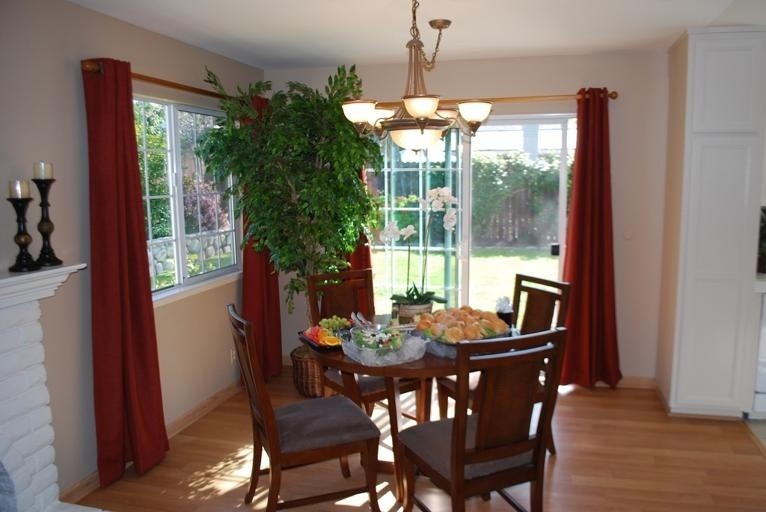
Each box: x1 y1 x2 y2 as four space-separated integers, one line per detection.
318 314 352 332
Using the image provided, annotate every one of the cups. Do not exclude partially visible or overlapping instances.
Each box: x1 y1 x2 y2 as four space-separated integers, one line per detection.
496 312 513 338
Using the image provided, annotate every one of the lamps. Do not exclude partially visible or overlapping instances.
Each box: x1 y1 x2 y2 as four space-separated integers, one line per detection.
340 0 495 155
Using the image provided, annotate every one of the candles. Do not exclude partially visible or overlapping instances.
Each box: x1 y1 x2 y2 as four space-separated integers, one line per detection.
33 160 53 180
9 178 30 198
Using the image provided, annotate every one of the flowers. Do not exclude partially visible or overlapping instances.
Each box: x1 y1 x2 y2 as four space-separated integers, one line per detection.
393 184 458 298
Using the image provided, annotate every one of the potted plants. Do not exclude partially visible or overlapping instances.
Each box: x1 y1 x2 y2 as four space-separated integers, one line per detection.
191 63 387 400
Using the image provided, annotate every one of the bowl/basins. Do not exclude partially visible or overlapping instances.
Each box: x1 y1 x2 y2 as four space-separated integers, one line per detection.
349 324 408 353
